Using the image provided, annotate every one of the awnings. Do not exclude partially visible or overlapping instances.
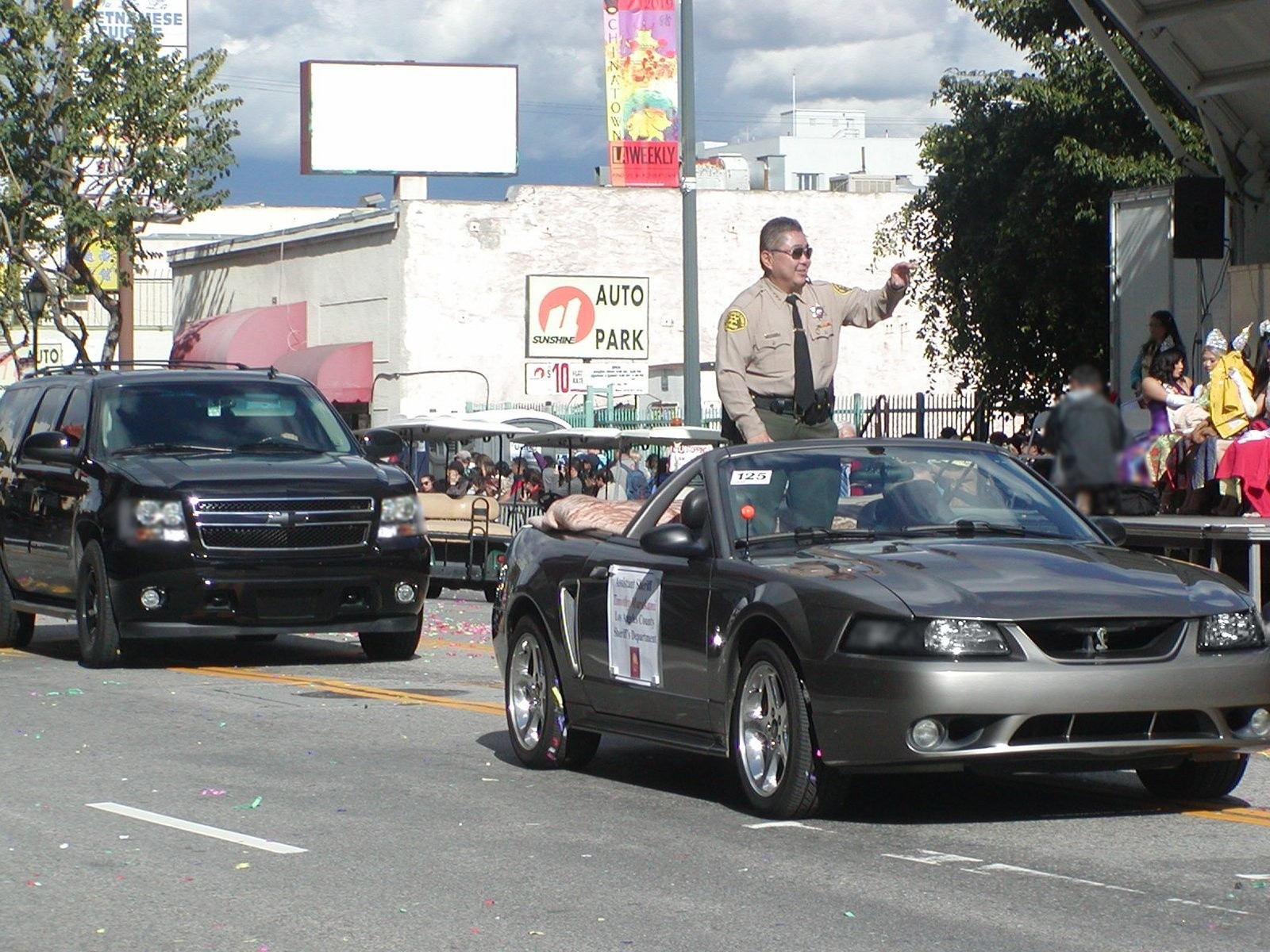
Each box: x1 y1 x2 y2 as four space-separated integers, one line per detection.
169 302 307 373
272 342 373 403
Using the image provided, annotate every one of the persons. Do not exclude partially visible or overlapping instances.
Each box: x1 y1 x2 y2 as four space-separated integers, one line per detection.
1116 347 1196 516
834 421 1052 511
421 446 671 509
1184 319 1270 518
1045 364 1128 514
1130 310 1189 411
715 217 919 540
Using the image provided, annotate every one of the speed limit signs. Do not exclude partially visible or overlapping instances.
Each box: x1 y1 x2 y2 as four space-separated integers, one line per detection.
525 358 650 395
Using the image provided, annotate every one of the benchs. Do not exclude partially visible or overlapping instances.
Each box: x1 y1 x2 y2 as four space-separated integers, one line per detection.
417 493 511 538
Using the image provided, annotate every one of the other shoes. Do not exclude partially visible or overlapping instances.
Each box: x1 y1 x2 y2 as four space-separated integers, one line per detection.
1213 496 1229 514
1220 499 1243 516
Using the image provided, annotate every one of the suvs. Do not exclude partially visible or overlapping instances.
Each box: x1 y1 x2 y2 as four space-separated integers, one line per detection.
1 360 432 669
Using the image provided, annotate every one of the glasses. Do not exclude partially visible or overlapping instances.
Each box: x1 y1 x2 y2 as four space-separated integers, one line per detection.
767 247 812 260
421 481 430 486
1149 323 1164 327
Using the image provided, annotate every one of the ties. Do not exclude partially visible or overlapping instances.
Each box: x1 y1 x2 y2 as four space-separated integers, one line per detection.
785 295 815 411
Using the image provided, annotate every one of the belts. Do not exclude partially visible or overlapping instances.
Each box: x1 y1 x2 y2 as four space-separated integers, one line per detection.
752 394 833 417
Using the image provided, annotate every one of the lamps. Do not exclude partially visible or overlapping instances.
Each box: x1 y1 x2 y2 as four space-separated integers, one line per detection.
363 192 385 208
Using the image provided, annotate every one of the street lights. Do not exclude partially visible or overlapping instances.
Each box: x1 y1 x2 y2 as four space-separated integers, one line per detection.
19 269 50 375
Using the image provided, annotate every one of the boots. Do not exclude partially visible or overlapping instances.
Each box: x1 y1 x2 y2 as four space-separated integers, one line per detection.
1177 487 1206 514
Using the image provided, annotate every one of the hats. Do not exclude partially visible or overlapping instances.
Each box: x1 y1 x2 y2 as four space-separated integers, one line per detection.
445 461 464 475
453 450 471 460
583 454 598 466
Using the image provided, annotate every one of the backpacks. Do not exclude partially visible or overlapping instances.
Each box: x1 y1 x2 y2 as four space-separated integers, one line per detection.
621 462 651 500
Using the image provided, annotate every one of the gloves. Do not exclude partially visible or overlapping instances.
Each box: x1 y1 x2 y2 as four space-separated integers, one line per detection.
1228 366 1258 418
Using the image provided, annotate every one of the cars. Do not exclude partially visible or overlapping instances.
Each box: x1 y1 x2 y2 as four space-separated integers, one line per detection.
348 409 733 601
489 437 1270 820
